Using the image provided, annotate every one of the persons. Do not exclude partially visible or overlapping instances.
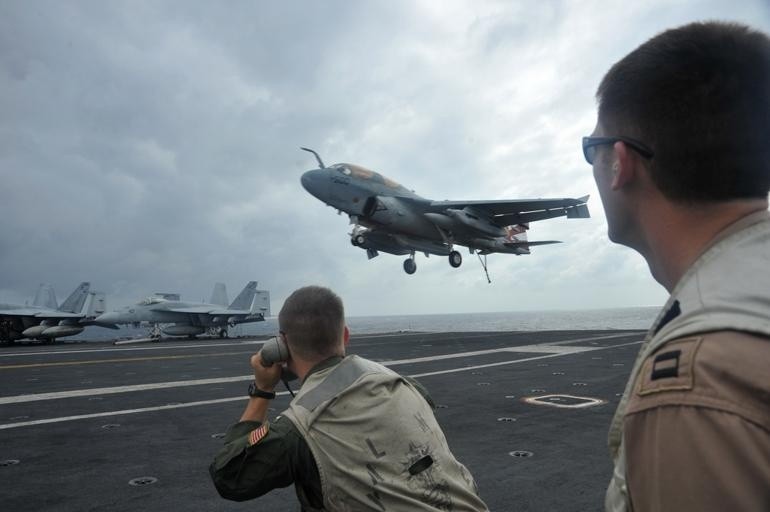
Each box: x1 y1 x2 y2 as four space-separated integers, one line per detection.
209 286 491 512
583 22 770 512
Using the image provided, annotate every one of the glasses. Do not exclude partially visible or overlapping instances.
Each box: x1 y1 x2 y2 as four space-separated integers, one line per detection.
582 136 654 164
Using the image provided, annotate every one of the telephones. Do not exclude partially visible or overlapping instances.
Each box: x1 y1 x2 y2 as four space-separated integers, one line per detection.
260 336 299 382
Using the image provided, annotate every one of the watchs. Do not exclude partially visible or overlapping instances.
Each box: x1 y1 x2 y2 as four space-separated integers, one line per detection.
248 382 275 399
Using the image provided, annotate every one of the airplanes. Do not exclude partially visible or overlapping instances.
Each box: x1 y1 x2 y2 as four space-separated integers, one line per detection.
300 148 589 282
0 281 270 346
